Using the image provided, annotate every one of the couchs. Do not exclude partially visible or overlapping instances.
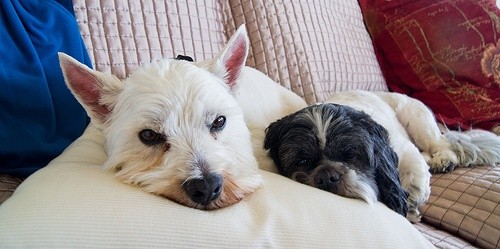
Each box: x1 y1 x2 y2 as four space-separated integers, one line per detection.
0 0 500 249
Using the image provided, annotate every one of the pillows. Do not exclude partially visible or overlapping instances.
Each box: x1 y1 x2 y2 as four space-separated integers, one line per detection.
0 119 437 249
358 0 500 130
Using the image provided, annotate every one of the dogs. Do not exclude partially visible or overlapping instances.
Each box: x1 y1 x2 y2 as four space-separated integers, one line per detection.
58 22 308 212
262 89 500 224
